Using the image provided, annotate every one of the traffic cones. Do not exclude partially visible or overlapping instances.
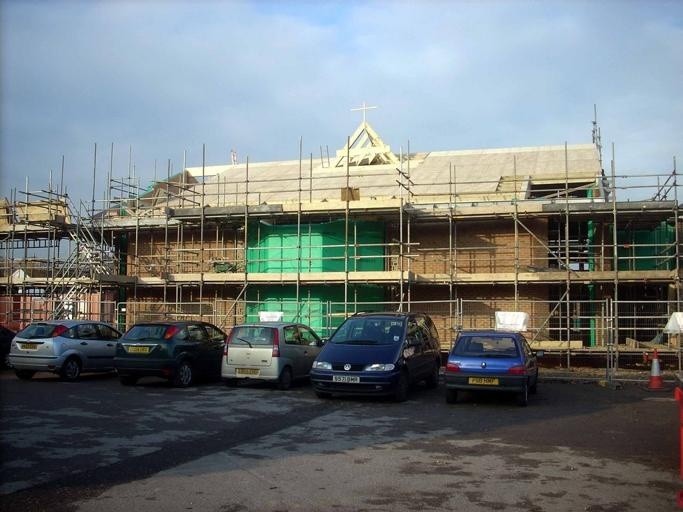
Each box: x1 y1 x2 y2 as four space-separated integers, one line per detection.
648 348 664 389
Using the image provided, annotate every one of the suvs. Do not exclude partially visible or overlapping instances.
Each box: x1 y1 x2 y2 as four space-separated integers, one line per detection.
309 310 442 404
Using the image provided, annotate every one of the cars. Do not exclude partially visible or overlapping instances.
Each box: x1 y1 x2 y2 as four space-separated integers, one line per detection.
9 320 122 383
220 322 328 391
112 320 229 389
443 328 544 407
